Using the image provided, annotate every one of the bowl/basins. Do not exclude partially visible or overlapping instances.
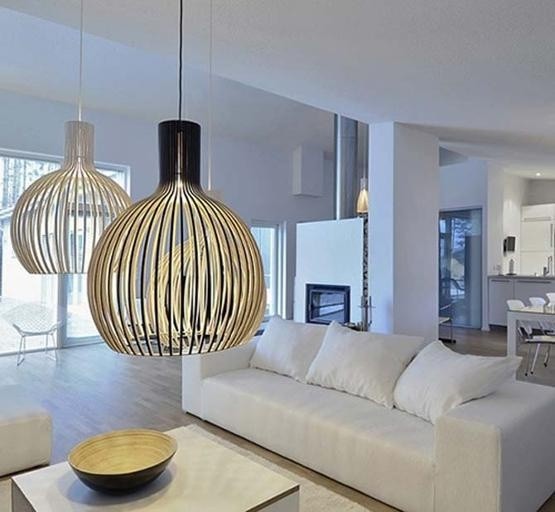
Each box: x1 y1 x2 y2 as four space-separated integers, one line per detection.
65 426 182 496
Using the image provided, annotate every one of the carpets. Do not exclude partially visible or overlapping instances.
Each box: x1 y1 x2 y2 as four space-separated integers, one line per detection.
0 423 371 511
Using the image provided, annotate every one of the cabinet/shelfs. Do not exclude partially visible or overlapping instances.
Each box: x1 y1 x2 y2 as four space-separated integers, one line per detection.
488 276 554 333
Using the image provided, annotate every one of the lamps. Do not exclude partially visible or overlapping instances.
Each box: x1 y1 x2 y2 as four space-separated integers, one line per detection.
355 123 369 214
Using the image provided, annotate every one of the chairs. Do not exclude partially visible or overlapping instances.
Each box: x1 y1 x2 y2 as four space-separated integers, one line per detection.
506 292 554 377
1 302 66 365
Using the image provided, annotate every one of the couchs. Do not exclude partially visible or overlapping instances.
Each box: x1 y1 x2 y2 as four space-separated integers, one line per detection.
181 314 555 511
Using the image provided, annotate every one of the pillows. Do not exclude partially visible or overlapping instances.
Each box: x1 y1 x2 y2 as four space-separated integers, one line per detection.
249 313 523 427
